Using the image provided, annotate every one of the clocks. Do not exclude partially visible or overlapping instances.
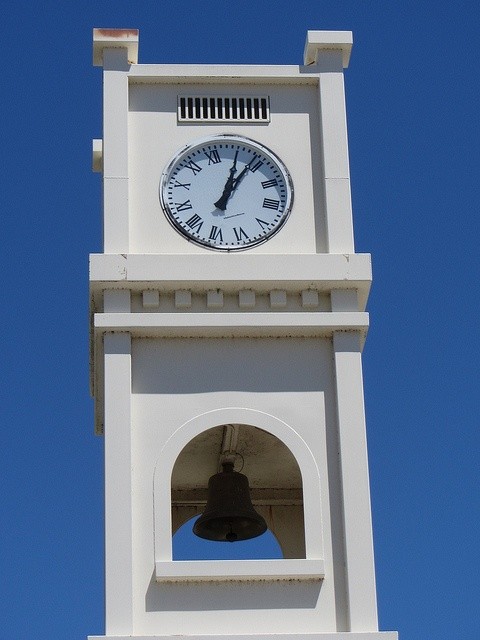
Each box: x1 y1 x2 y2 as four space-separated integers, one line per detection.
158 134 294 253
